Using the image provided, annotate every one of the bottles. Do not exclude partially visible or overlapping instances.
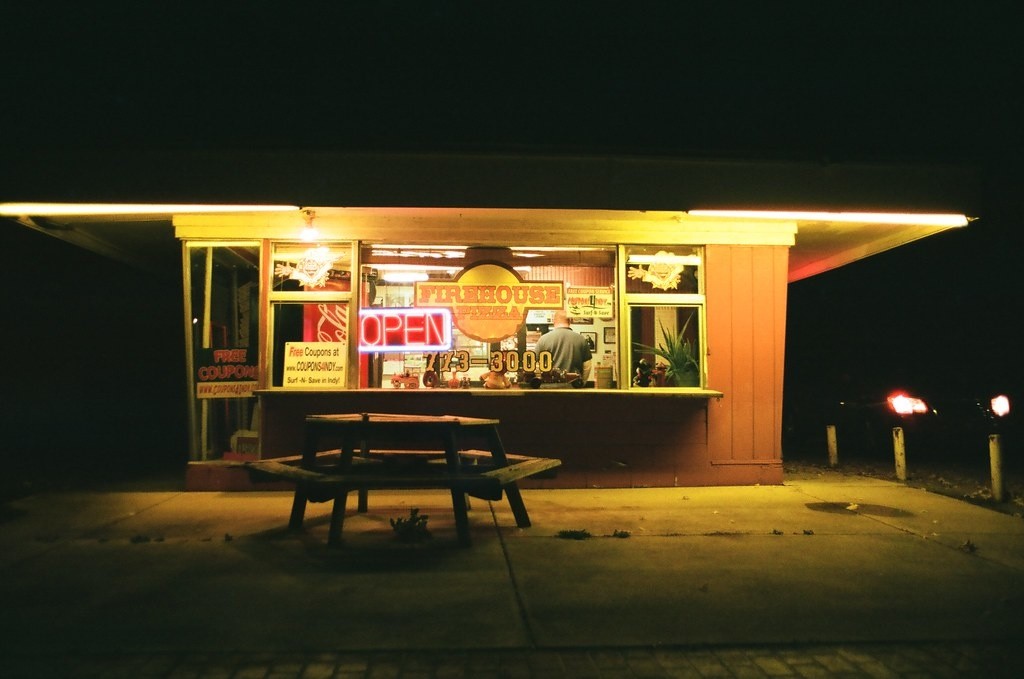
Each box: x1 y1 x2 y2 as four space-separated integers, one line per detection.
448 372 472 389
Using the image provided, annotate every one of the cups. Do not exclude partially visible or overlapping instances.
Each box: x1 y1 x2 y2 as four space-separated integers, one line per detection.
594 365 613 389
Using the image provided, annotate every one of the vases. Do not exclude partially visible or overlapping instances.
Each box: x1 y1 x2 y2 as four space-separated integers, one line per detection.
447 371 461 388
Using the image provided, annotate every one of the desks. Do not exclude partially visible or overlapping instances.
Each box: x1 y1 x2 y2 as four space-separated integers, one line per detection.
244 410 532 547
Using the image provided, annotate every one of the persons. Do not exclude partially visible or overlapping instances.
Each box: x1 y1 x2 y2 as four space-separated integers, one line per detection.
534 311 588 388
406 370 410 377
461 376 471 388
480 361 511 389
585 335 594 349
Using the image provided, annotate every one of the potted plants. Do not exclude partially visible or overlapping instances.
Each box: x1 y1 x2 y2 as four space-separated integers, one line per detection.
632 312 700 387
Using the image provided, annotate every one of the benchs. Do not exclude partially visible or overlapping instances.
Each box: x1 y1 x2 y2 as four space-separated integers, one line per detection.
228 447 563 494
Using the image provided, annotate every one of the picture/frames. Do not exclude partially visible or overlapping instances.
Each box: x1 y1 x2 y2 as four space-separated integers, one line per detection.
603 327 616 344
580 332 597 353
571 317 593 325
